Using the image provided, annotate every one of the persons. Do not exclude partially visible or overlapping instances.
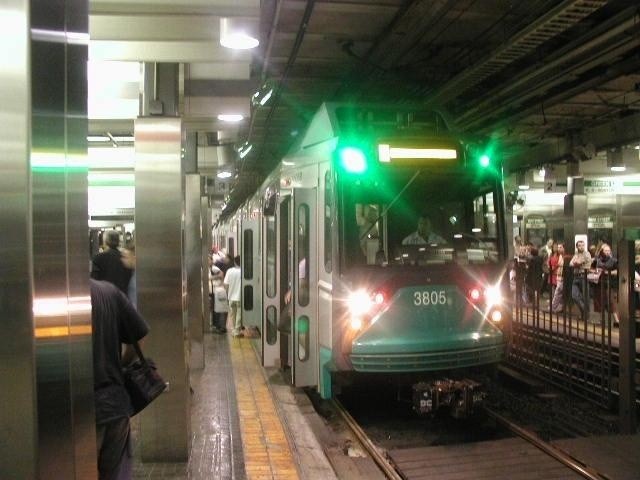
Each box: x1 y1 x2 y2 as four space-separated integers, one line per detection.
283 234 307 352
355 204 382 260
510 234 639 338
89 229 248 337
88 277 152 479
401 214 447 246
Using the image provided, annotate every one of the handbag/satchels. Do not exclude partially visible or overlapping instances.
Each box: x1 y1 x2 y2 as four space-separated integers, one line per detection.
121 357 167 419
588 272 602 284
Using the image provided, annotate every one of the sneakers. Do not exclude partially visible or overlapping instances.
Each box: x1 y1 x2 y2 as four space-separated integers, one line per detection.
211 324 244 337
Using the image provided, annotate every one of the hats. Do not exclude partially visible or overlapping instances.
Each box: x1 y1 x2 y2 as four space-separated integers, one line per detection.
103 230 119 245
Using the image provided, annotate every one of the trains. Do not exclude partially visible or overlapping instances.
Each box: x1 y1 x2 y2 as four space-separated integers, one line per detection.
207 98 521 420
